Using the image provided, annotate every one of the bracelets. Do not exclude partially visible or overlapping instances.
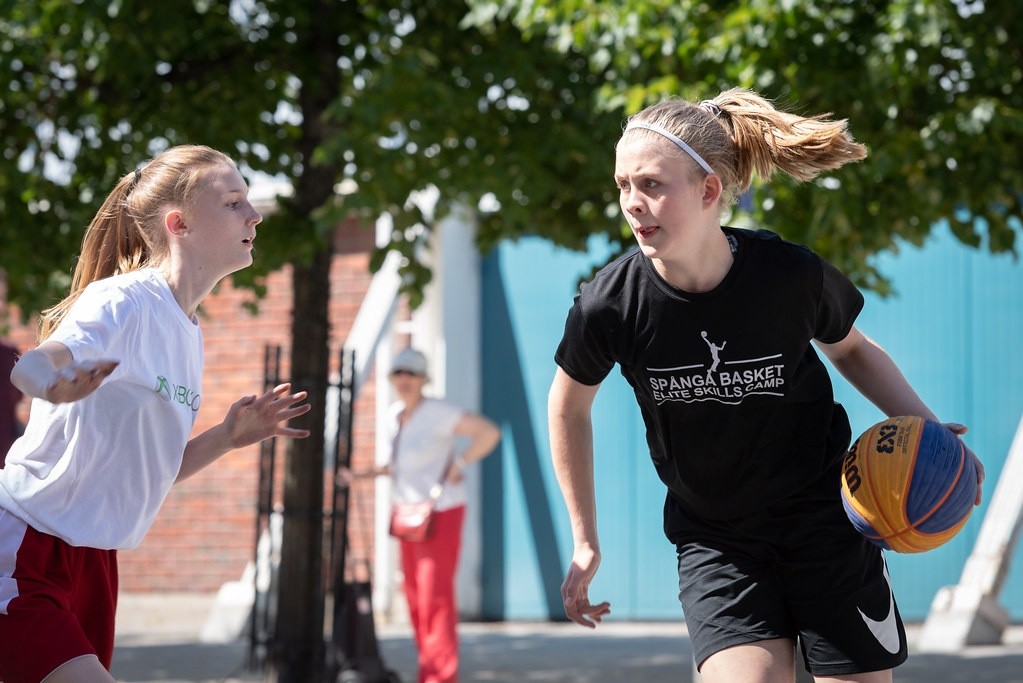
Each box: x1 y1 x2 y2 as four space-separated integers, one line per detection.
454 454 465 470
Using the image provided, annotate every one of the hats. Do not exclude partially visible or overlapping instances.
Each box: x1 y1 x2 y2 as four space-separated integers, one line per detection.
388 349 431 384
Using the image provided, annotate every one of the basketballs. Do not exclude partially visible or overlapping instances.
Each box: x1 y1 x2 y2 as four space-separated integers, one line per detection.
841 415 979 554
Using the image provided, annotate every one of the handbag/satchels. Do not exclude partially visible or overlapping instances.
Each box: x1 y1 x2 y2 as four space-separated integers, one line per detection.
389 501 434 543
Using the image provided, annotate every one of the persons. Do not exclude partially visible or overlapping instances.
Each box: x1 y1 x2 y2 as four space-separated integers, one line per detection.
335 348 500 683
546 86 985 683
0 143 311 683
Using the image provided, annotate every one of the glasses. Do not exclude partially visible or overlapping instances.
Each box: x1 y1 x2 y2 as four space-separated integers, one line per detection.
393 369 416 376
